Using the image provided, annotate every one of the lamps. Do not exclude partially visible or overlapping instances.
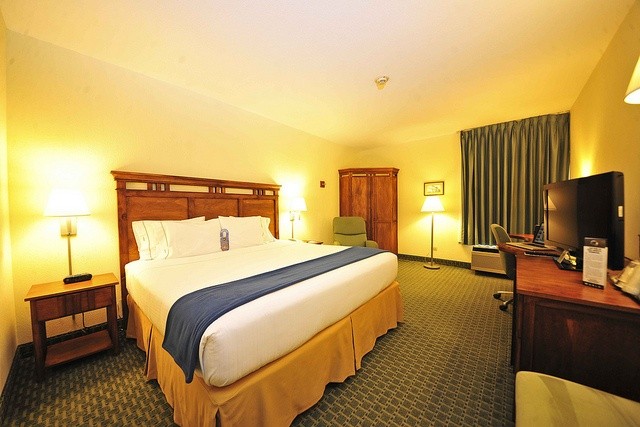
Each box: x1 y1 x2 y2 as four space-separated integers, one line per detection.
43 190 92 277
624 56 640 105
420 196 445 270
285 198 307 239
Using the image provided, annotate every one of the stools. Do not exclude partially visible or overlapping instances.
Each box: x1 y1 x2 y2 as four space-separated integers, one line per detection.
513 371 640 427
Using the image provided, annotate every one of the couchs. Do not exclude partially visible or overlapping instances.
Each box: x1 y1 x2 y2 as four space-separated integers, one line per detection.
332 216 379 249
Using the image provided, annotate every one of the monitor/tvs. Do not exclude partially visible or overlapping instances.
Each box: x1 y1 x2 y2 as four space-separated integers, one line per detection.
542 179 584 257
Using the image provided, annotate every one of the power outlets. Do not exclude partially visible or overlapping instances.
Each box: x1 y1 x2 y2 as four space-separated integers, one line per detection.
61 217 77 236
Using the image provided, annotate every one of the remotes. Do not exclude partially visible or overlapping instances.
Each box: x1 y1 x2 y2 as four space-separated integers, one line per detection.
64 272 94 284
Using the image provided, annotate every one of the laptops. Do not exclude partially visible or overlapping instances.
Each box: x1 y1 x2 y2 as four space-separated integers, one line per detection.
507 220 550 251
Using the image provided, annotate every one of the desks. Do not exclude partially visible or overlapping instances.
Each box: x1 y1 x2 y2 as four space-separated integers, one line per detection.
496 241 563 256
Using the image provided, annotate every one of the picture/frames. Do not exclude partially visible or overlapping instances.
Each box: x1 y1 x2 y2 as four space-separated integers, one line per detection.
424 181 444 196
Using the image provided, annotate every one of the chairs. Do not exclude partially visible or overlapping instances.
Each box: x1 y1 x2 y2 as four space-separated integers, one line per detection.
490 223 517 311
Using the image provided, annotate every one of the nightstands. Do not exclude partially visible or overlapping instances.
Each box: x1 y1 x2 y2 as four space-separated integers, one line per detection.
296 240 324 245
23 273 120 382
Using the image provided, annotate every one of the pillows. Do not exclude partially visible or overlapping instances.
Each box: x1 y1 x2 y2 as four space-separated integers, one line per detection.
218 215 262 249
162 219 221 260
132 216 206 261
229 215 276 243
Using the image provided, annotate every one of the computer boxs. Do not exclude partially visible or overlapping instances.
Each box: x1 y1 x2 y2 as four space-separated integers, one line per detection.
583 171 625 270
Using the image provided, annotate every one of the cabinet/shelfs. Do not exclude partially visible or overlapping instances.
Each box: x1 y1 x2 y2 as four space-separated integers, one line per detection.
510 288 640 402
338 168 400 256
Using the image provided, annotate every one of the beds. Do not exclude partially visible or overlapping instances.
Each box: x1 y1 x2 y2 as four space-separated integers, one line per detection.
111 170 404 427
